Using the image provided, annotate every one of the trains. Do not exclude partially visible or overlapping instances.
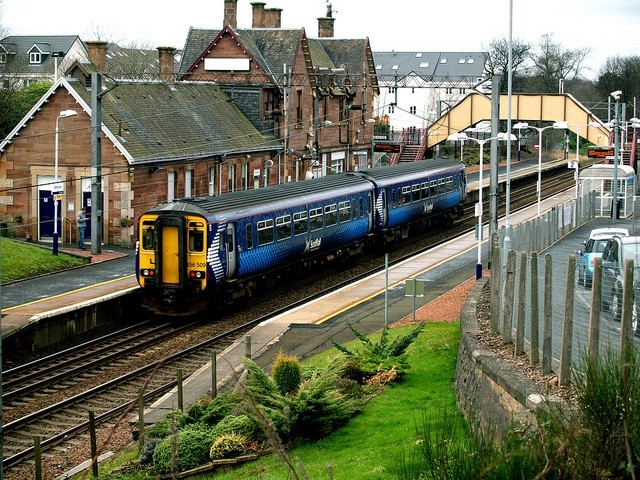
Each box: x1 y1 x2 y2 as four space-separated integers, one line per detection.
135 156 467 316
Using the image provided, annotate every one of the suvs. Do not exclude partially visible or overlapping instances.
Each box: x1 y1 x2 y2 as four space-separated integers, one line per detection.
599 235 640 321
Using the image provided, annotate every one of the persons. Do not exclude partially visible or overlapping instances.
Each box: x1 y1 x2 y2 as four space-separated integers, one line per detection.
77 207 90 249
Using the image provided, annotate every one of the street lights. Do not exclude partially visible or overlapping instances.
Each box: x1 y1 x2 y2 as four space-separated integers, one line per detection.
53 109 78 255
446 132 517 280
611 118 640 143
512 122 569 215
603 123 640 165
553 121 601 197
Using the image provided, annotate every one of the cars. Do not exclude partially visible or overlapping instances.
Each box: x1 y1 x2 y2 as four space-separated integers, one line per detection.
577 235 624 288
581 228 629 246
631 281 640 336
